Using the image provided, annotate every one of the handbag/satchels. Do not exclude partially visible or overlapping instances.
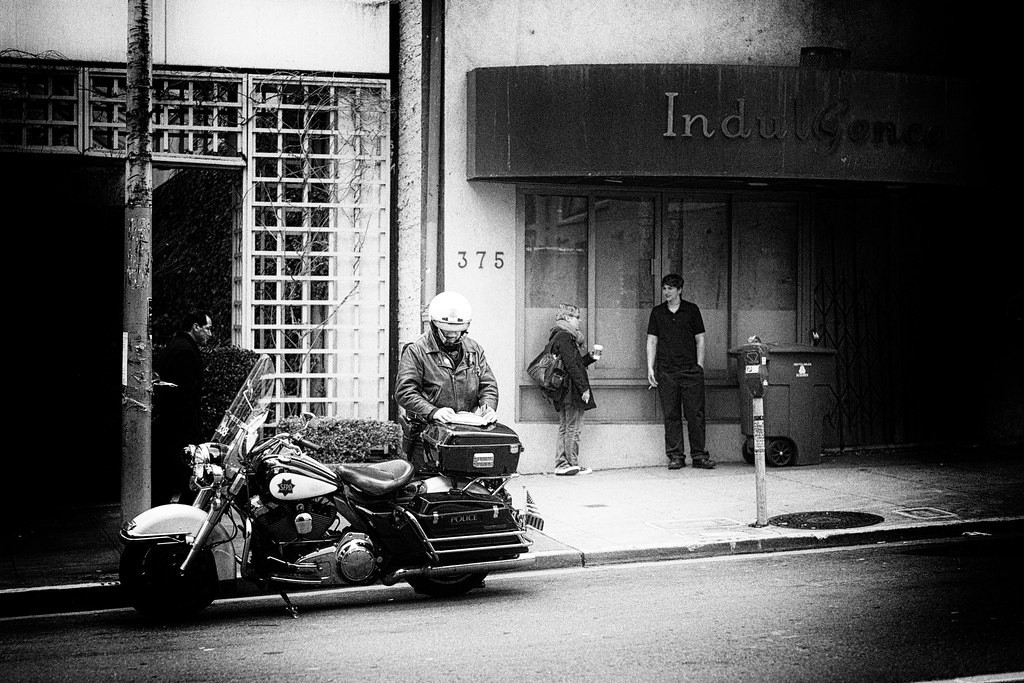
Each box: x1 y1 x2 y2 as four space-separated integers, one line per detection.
527 331 572 391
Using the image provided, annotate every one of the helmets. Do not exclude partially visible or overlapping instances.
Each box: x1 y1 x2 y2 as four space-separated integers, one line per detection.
428 291 472 331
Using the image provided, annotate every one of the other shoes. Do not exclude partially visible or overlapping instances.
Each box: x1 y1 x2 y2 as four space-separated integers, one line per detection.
692 458 716 468
573 465 593 475
555 464 580 476
668 458 686 469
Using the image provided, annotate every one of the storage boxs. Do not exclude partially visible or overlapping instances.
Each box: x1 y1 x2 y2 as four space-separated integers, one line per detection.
410 495 520 552
421 424 523 477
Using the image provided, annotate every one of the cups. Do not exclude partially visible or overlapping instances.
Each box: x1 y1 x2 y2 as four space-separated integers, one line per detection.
593 345 603 360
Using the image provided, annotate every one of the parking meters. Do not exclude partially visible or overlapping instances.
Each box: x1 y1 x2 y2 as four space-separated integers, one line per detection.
741 341 770 527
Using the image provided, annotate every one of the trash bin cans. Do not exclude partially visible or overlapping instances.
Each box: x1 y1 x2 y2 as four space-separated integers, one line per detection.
728 342 840 467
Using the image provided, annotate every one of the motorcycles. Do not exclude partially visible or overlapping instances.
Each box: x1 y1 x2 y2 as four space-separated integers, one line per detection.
115 354 537 626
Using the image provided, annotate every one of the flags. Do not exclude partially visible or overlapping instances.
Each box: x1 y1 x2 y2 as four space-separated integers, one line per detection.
525 492 544 531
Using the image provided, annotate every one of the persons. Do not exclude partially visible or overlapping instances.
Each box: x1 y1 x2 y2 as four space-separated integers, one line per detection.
151 312 213 505
549 303 603 475
646 273 716 469
394 291 499 478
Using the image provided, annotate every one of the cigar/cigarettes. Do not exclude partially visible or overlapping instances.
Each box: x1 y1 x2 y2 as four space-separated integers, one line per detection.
648 383 654 389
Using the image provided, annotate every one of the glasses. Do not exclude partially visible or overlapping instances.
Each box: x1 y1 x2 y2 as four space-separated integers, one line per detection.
569 314 581 319
